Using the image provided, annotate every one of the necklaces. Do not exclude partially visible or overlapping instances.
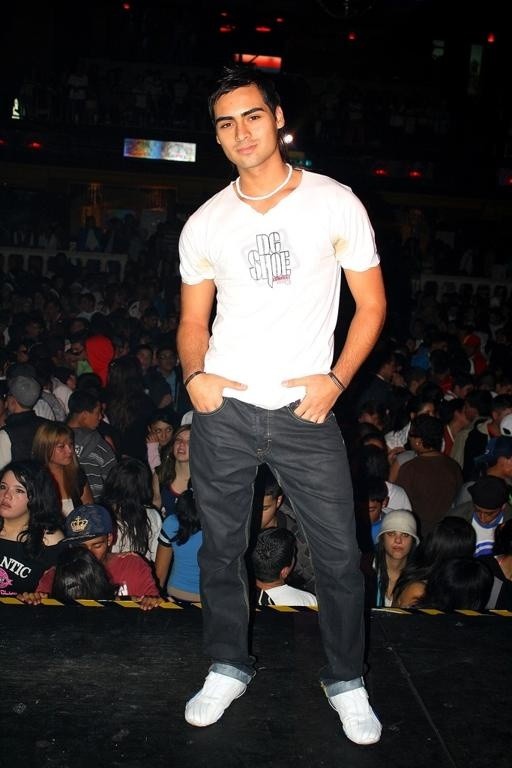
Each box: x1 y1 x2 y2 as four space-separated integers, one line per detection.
234 163 291 201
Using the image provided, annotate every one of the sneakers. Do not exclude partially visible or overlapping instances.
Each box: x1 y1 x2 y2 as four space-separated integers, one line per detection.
184 670 248 727
328 686 383 745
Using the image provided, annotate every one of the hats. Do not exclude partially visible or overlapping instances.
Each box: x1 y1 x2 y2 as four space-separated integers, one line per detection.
8 375 41 406
62 503 113 541
377 511 421 545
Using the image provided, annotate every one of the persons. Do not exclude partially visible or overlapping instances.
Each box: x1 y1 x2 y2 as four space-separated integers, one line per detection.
174 64 387 748
1 206 510 613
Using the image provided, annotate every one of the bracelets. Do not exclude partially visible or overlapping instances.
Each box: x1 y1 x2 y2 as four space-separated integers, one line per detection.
327 372 347 393
183 370 207 386
